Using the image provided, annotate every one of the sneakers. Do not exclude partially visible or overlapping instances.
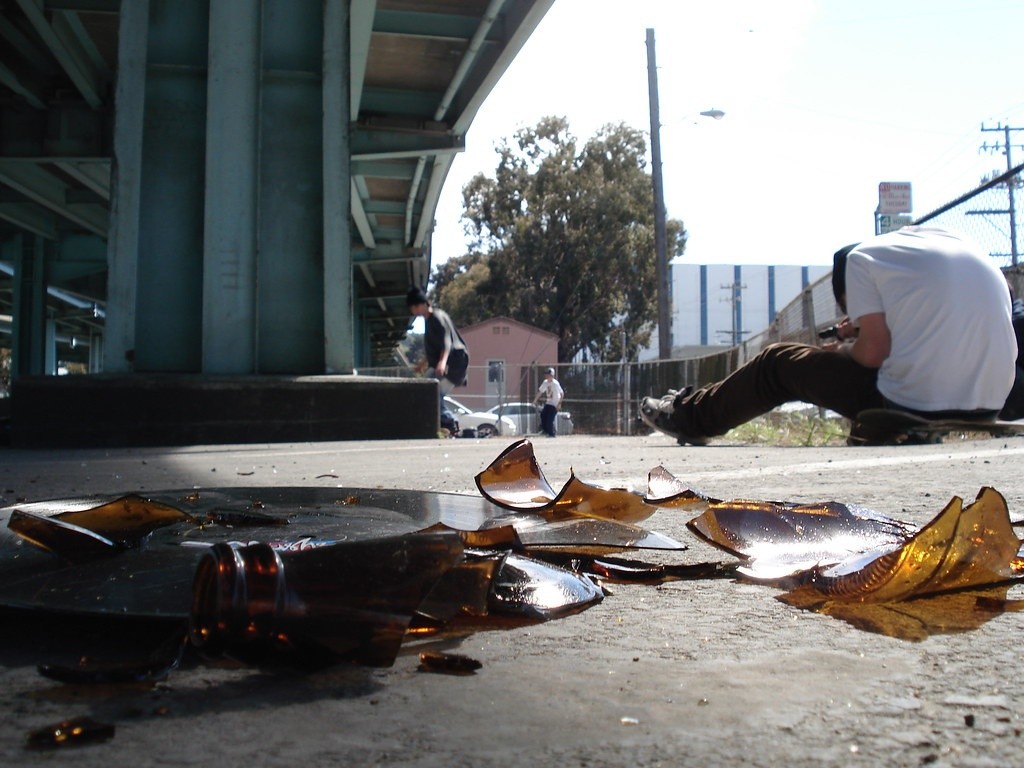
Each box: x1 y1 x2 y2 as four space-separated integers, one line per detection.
640 384 712 446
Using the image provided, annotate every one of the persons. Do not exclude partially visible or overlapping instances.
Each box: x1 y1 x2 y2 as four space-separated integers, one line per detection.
531 368 563 436
640 227 1017 446
407 287 469 433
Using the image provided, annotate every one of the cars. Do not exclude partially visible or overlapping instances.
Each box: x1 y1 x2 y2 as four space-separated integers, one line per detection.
444 396 516 437
484 403 574 435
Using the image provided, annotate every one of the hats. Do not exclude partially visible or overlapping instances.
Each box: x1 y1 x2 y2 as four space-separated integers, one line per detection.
406 287 428 304
543 367 555 376
